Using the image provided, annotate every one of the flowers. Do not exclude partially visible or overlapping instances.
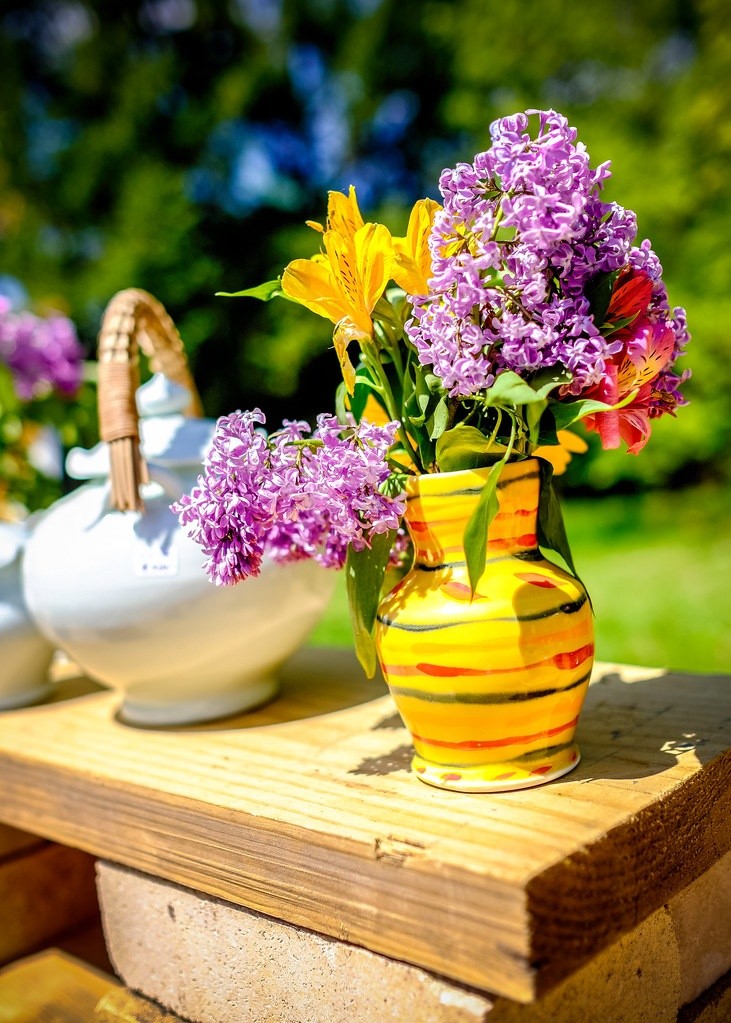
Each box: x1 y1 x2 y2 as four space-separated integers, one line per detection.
0 295 108 514
169 106 690 632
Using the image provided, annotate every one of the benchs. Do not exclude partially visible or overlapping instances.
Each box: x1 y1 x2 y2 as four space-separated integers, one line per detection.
0 646 731 1023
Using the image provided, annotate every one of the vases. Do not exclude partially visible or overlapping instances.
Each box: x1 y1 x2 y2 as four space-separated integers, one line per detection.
370 467 590 791
0 519 59 711
